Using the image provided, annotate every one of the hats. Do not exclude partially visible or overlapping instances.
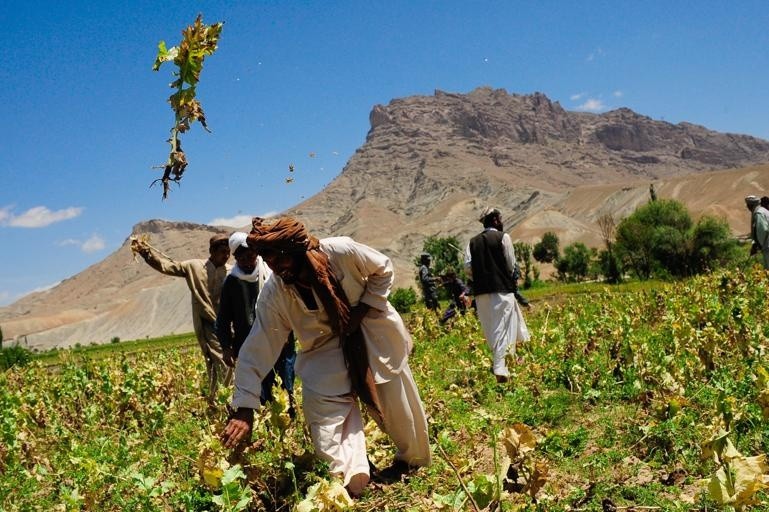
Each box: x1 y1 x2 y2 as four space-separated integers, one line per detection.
420 253 430 260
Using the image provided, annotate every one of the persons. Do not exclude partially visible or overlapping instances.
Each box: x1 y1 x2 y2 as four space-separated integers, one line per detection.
419 250 442 312
213 228 297 425
513 262 531 313
743 193 769 273
464 203 533 385
436 270 472 328
219 214 433 501
127 233 235 395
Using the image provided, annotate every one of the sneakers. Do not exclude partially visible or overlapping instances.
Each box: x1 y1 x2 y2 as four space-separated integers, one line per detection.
494 374 510 383
